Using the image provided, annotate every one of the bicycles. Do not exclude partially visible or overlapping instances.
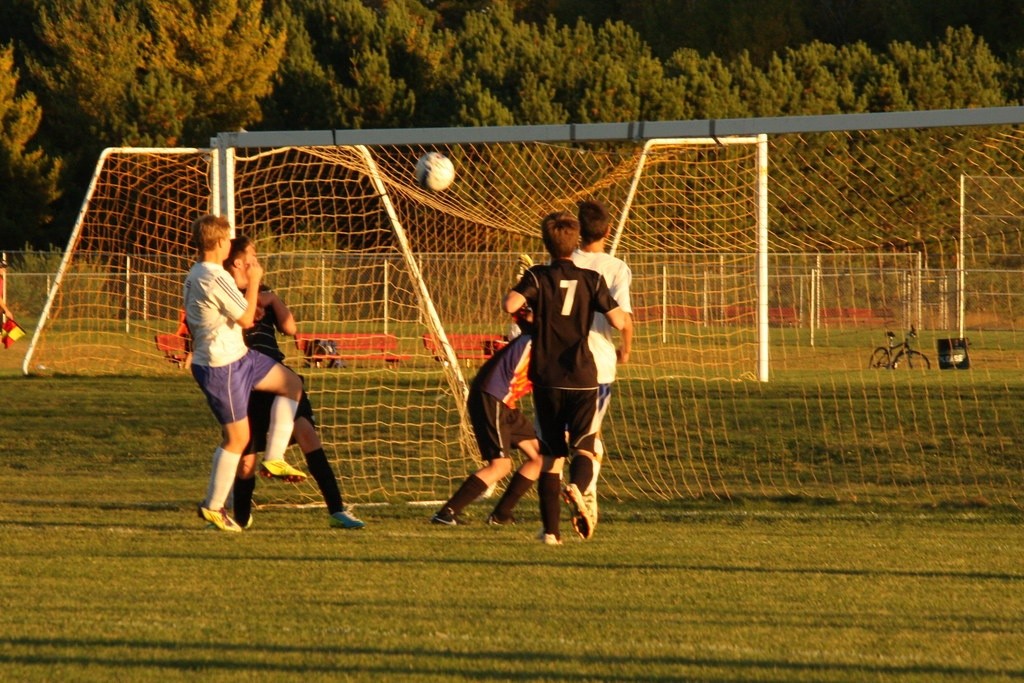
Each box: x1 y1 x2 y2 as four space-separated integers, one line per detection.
867 323 932 372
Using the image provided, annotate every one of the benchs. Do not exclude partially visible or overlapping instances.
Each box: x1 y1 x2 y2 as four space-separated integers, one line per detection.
423 330 512 364
155 332 189 370
294 330 412 368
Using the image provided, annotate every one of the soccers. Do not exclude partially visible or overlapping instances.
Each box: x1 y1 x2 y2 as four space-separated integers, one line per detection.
414 151 456 191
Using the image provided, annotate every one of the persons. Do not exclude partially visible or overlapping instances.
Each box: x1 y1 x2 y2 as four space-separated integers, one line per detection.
182 216 364 532
431 200 632 545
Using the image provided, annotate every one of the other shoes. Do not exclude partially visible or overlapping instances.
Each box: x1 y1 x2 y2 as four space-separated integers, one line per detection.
582 488 597 525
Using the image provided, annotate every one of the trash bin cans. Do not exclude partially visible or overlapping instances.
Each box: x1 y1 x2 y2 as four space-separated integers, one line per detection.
937 338 971 370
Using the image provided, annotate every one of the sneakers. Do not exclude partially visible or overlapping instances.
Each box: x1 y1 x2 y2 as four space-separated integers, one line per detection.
232 510 253 529
562 484 592 540
329 506 365 528
197 501 243 532
259 458 306 482
543 533 562 546
487 515 518 527
432 508 467 526
